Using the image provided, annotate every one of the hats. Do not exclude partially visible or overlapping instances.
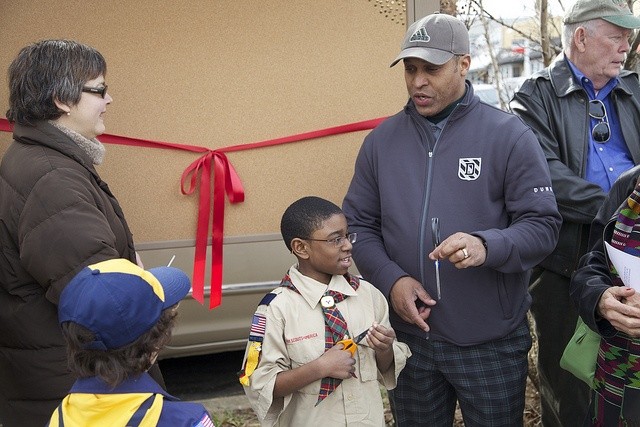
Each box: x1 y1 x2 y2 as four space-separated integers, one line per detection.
58 257 190 351
390 9 470 68
562 0 640 29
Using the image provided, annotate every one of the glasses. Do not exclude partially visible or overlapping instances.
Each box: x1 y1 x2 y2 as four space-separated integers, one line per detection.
296 232 358 248
588 99 611 143
80 84 108 97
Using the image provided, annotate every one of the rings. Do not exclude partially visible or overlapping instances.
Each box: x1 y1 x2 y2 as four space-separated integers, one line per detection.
462 248 470 258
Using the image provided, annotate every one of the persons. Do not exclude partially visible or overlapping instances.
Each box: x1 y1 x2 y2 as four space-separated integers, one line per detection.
571 165 640 425
239 197 412 427
509 0 640 425
47 258 216 426
340 12 560 427
0 41 168 426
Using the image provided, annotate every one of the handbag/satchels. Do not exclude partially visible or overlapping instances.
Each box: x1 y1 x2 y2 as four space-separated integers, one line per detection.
559 312 601 387
